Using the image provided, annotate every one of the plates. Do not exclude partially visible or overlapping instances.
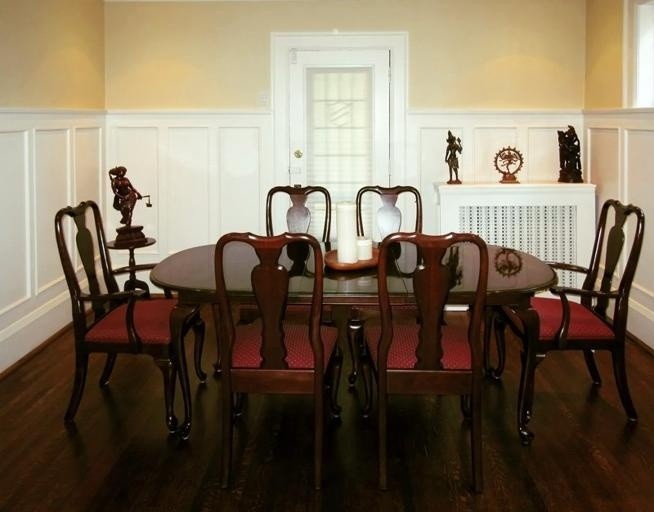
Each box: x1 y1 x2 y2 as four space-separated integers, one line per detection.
323 267 382 281
323 249 381 269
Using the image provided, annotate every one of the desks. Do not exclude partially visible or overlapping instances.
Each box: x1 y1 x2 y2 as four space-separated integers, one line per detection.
104 237 157 298
150 234 561 448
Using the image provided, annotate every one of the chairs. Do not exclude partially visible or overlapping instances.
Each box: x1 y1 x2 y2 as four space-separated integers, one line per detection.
210 226 347 512
353 231 500 506
494 191 648 448
348 175 433 393
47 199 212 440
261 178 338 329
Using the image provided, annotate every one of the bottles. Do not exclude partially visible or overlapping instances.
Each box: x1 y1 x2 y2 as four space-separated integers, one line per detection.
335 201 356 263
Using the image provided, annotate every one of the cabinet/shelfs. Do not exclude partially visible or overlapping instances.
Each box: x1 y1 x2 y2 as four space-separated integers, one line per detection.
432 177 598 309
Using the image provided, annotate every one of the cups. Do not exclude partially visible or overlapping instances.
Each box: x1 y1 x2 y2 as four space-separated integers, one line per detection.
357 236 374 259
356 276 371 292
